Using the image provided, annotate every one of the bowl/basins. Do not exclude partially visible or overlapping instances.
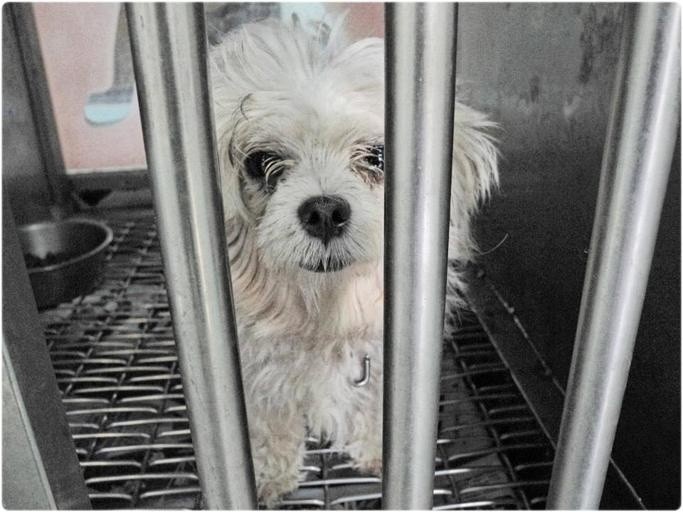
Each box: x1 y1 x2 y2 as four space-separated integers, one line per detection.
13 218 114 312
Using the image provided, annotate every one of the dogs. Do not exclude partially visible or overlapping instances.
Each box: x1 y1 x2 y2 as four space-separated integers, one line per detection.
207 1 503 509
